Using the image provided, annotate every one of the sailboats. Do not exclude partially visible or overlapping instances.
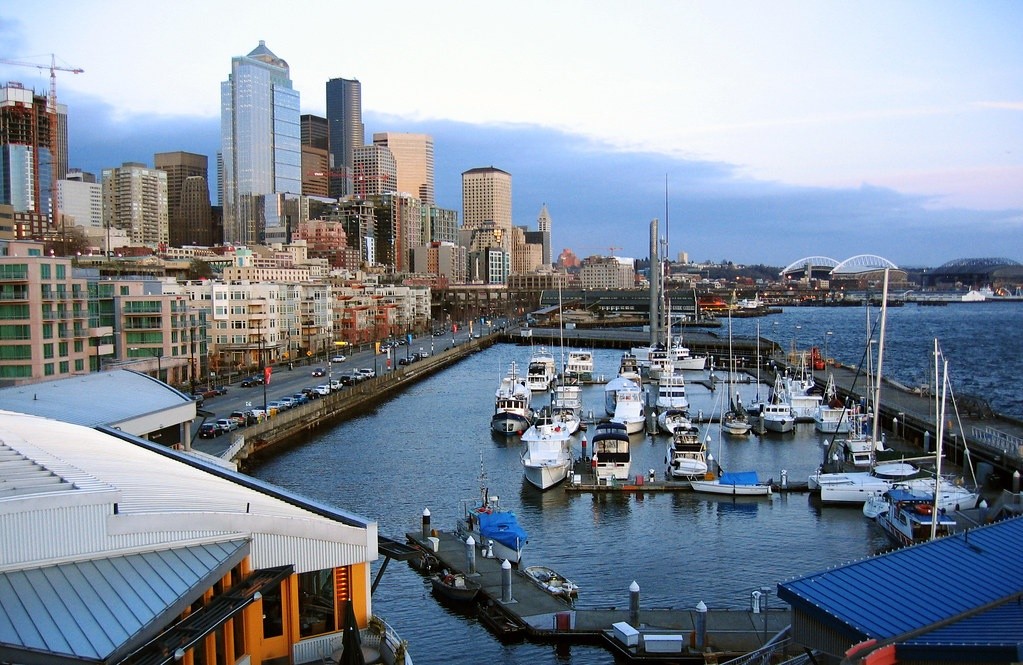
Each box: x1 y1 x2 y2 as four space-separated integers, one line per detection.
807 265 949 501
867 359 959 548
720 309 752 435
687 379 772 495
862 336 981 519
549 276 581 432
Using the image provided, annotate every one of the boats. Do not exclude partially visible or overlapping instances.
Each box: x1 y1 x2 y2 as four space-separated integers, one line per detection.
812 370 853 433
526 297 826 436
524 566 578 595
519 406 573 491
609 391 645 433
664 396 707 478
764 373 797 432
431 570 482 600
593 422 633 480
457 447 528 564
491 359 534 433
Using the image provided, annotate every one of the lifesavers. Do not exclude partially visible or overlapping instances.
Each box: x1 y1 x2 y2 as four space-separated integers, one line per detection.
445 574 455 585
914 504 933 514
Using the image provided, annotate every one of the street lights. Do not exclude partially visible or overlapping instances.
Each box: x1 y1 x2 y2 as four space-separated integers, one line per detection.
96 331 122 372
131 347 162 381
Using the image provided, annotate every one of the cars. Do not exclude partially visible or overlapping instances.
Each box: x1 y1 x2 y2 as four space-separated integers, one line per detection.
190 324 462 441
